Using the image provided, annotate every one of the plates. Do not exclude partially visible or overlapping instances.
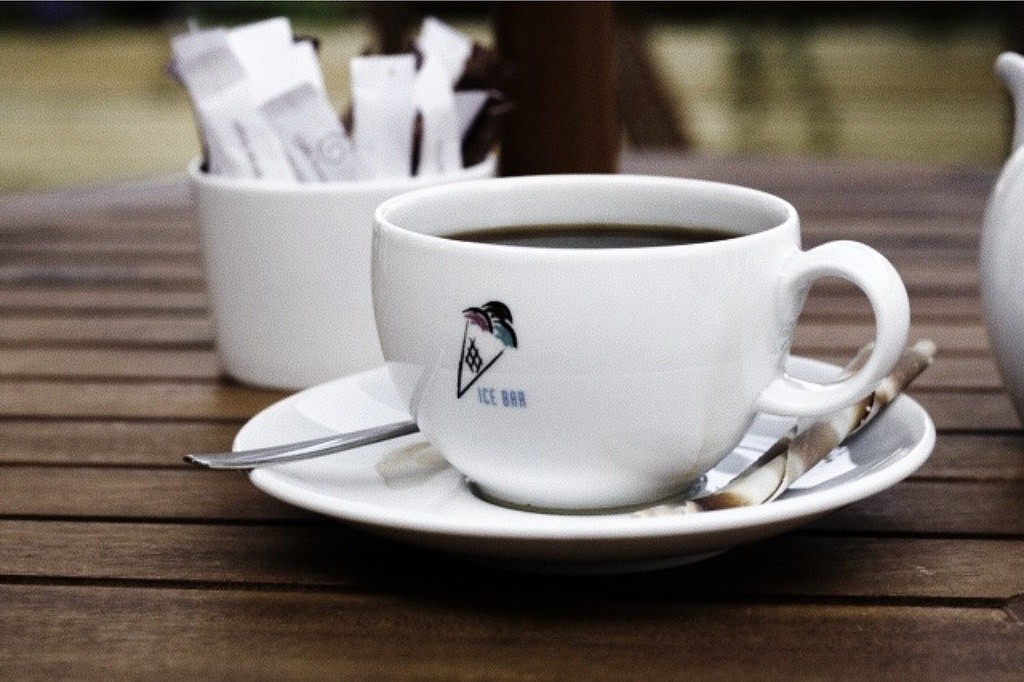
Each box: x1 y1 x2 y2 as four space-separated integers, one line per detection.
232 352 936 575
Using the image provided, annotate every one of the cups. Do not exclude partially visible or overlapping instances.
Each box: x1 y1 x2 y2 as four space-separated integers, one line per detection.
371 171 912 515
187 150 500 395
